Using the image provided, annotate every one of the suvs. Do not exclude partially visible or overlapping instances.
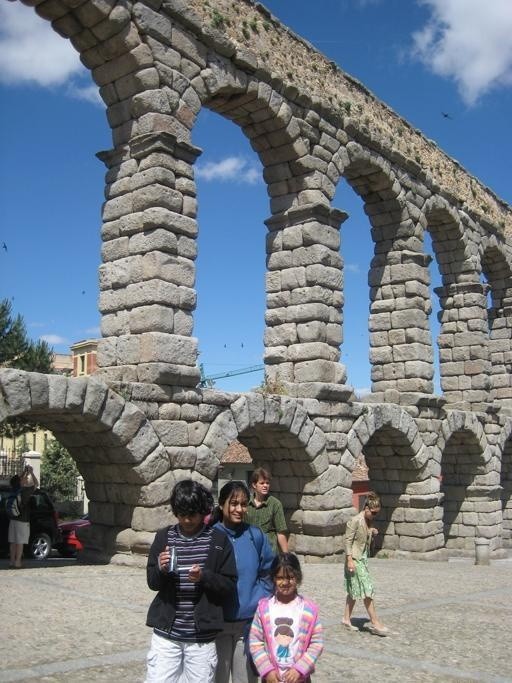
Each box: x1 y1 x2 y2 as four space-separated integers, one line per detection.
0 479 60 560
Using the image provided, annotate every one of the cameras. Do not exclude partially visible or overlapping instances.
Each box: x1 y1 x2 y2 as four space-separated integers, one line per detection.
167 546 192 575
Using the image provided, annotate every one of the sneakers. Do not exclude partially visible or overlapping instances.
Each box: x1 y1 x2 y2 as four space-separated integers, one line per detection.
340 620 360 631
369 624 392 634
9 564 25 570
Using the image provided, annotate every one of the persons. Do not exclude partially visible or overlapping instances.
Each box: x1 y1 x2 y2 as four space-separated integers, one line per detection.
338 490 390 637
241 466 289 556
207 482 276 682
6 464 38 568
144 479 238 683
247 552 323 683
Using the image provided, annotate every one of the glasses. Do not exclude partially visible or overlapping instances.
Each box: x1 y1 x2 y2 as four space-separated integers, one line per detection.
369 509 378 515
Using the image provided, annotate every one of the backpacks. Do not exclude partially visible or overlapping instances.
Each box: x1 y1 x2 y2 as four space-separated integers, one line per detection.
4 487 24 518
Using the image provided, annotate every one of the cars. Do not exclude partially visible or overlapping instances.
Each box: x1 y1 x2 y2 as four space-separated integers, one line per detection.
52 513 92 556
